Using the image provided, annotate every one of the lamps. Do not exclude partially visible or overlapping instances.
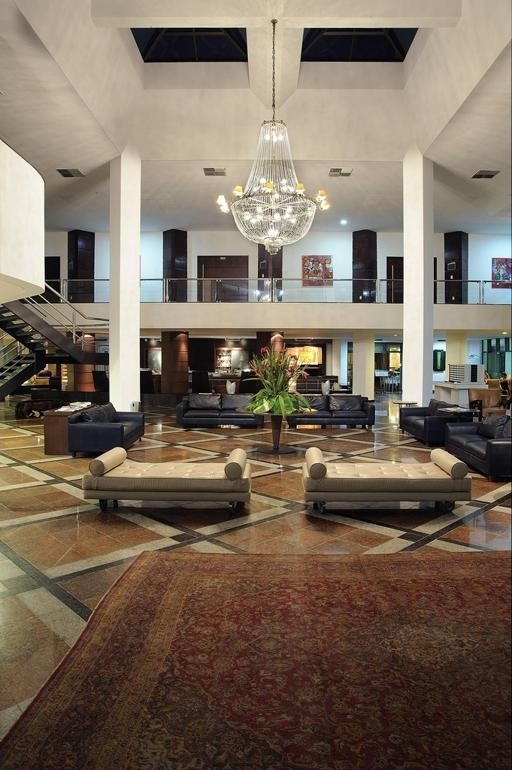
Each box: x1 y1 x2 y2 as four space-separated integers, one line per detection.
216 0 332 256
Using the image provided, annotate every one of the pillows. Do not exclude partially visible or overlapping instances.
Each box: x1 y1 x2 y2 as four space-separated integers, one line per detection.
81 402 120 424
428 399 453 416
302 394 363 412
189 393 255 412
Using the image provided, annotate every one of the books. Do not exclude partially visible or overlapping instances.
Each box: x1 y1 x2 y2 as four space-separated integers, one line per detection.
54 401 91 413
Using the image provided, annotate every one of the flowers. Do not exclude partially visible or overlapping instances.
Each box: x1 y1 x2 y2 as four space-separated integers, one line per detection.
243 342 314 414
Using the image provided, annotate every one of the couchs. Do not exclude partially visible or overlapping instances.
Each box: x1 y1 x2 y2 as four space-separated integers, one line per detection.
399 405 512 482
175 392 265 430
286 393 375 432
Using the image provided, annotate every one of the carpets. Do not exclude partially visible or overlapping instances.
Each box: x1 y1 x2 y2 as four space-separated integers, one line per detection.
1 549 512 770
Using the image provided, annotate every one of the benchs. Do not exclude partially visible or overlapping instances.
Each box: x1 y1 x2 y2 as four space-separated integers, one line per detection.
301 447 474 517
80 447 252 517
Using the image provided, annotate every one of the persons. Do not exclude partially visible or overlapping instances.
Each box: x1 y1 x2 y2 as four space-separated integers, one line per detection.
484 370 491 383
499 372 511 409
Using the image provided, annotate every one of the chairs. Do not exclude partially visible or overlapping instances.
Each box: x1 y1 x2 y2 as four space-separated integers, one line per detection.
64 403 145 457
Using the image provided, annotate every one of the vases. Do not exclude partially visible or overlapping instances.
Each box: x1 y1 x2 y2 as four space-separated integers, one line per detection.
270 408 282 451
